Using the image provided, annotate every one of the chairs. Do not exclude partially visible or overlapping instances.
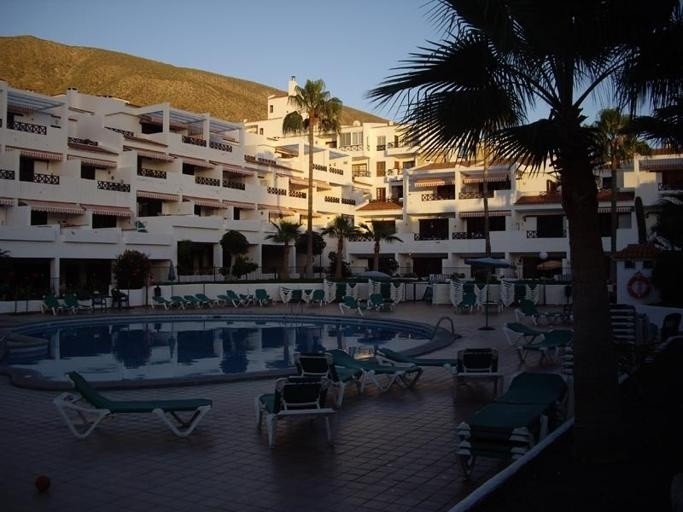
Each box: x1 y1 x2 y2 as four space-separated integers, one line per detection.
254 342 499 447
419 279 543 313
608 304 682 361
54 371 213 441
37 288 129 316
279 276 405 318
503 298 573 371
152 288 274 311
452 371 569 481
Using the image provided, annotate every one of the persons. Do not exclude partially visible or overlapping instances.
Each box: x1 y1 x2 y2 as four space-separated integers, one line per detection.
111 284 123 311
59 281 68 299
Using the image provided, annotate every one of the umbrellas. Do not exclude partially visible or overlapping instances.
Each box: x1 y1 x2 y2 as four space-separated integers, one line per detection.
462 256 516 328
168 261 176 297
353 270 393 311
